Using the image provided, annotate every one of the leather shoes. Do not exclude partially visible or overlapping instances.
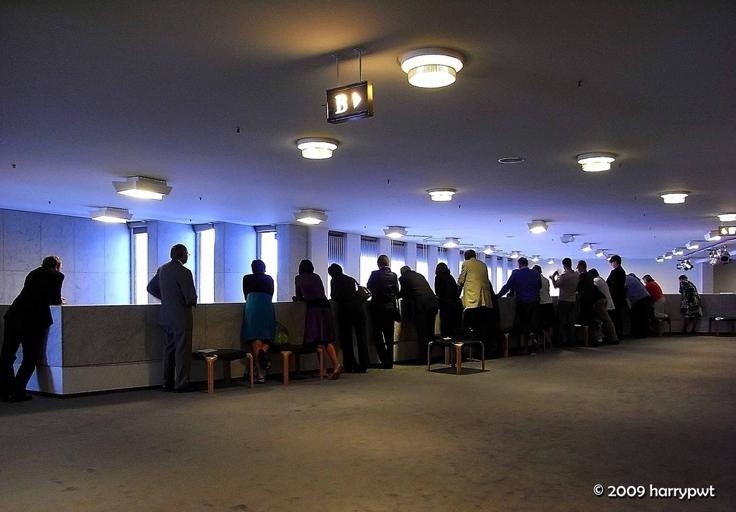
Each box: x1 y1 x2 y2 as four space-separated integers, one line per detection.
331 363 343 379
313 372 329 377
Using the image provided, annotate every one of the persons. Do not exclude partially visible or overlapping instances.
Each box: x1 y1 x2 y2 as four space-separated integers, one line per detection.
0 255 64 403
398 265 438 364
291 259 344 380
434 263 467 362
678 274 705 335
328 263 369 373
367 254 399 369
243 260 276 384
146 243 197 393
456 249 494 328
496 255 671 356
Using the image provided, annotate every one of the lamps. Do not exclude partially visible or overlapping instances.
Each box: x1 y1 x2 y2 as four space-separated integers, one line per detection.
655 213 735 271
296 137 339 160
576 151 615 173
111 173 174 201
292 208 328 226
660 191 689 205
440 234 612 265
383 225 409 240
426 188 457 202
528 217 548 234
90 207 134 224
398 46 465 90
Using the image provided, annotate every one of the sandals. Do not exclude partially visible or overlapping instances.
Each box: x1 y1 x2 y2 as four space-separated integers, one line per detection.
250 378 265 384
258 349 266 369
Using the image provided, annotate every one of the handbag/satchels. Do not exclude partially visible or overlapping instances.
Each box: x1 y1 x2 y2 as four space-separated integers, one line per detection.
351 277 372 299
273 321 288 345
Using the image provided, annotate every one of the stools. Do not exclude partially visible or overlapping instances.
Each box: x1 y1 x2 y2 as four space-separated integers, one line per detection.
707 315 735 336
193 349 255 393
653 312 674 336
500 327 525 359
427 333 487 373
273 344 326 385
